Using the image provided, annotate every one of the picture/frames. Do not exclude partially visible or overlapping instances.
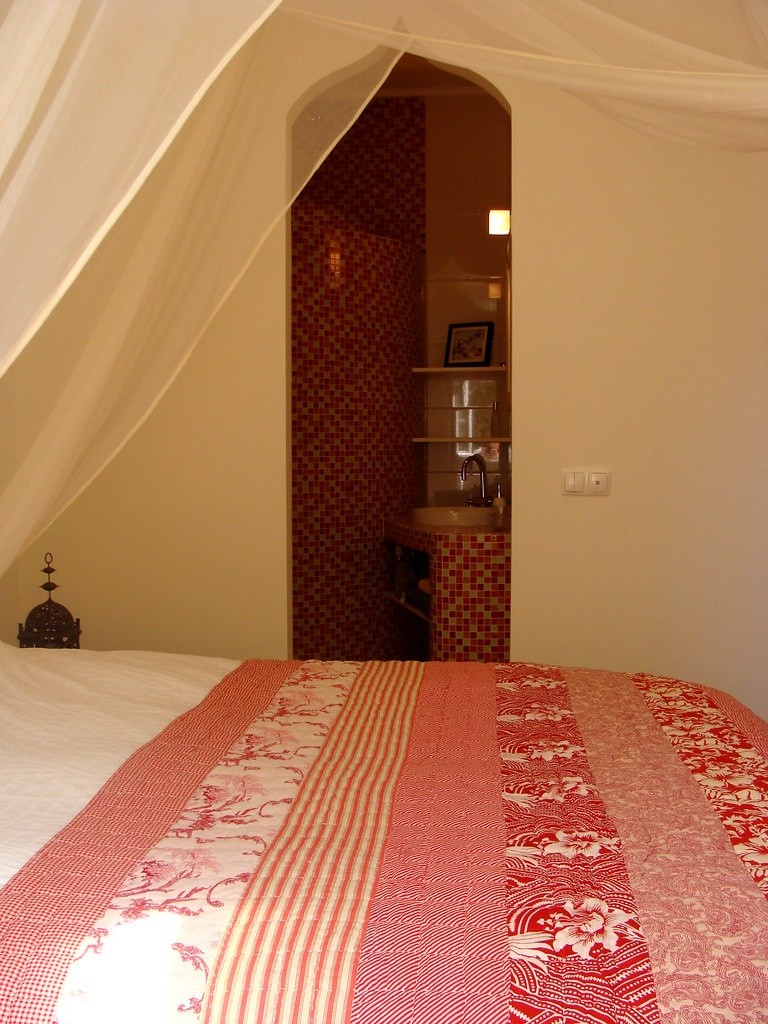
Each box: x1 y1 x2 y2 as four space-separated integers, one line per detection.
443 321 494 366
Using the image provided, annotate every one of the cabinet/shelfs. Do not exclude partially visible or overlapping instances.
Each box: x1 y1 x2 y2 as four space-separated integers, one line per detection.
409 365 510 444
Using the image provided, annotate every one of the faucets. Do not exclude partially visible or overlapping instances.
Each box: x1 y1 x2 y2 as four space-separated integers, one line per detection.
460 454 493 507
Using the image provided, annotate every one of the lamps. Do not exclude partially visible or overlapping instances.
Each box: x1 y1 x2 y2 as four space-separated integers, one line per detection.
488 209 511 235
488 281 502 298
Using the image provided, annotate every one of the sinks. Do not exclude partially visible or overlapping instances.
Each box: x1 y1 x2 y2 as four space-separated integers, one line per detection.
411 506 494 526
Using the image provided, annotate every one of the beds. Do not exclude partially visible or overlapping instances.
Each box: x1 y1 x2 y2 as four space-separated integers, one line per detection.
0 643 768 1024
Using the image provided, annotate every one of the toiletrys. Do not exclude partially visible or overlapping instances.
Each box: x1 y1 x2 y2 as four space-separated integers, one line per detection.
491 482 506 532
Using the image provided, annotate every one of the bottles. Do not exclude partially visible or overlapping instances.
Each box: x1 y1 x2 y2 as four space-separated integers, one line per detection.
491 497 506 532
491 401 500 438
